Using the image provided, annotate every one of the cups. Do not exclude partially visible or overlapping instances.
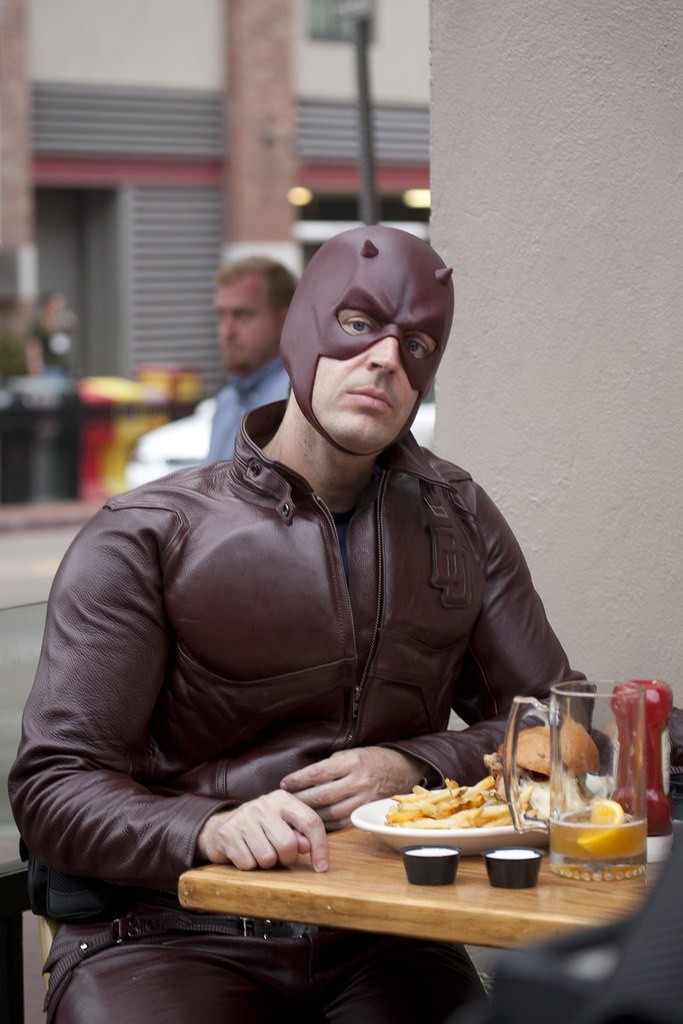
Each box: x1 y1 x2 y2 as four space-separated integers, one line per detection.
400 845 462 884
502 680 648 881
483 847 546 888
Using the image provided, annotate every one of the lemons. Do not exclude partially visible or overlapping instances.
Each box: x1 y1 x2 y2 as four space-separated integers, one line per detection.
578 798 625 853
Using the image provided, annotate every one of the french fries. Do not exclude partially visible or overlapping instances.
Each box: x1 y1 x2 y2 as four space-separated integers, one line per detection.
385 776 513 829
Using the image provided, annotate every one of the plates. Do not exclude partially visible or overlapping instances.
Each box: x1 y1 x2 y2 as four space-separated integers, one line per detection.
350 791 550 855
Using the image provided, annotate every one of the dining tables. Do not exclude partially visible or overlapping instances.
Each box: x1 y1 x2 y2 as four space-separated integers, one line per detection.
179 722 683 954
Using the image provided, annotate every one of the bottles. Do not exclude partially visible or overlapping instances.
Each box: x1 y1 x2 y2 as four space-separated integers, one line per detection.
611 682 674 861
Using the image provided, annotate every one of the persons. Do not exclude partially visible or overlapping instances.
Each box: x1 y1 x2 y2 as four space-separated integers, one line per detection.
24 288 79 507
7 226 591 1024
209 257 299 466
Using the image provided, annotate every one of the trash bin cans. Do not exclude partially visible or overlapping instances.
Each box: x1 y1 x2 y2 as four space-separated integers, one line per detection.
1 362 206 507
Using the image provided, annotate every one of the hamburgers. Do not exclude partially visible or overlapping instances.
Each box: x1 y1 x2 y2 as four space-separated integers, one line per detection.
483 718 600 825
